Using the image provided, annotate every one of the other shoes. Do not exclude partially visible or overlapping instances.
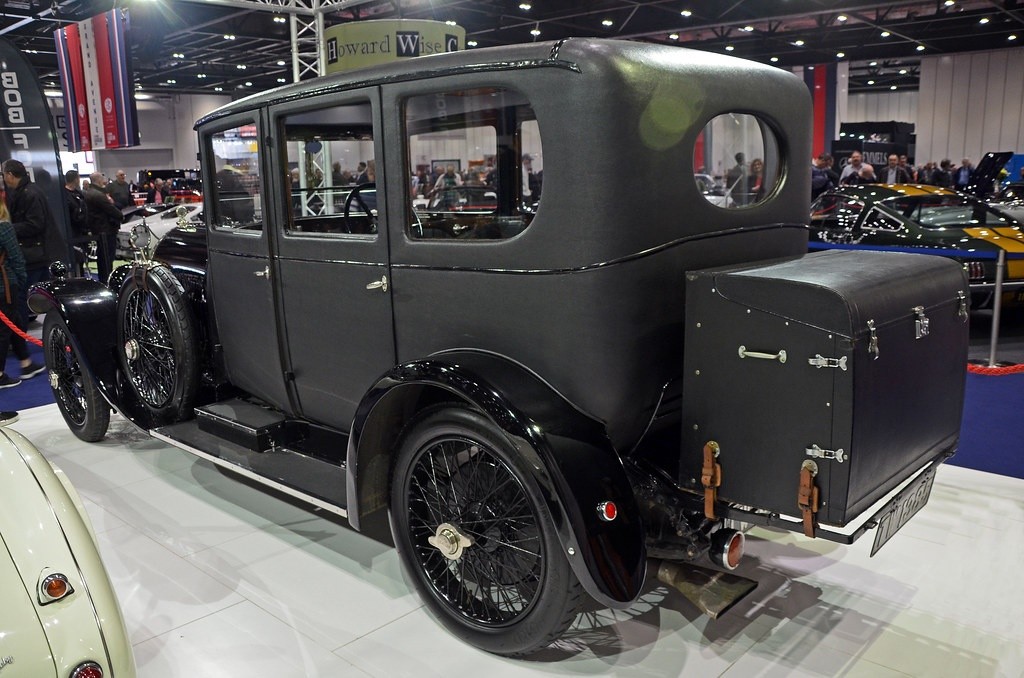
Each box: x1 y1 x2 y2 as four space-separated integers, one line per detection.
0 411 19 426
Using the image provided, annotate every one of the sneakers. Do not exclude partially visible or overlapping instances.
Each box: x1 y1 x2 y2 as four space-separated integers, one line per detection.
0 374 22 389
19 361 46 379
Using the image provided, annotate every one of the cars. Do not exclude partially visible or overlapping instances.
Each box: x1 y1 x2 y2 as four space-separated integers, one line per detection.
27 34 971 657
912 183 1024 232
810 185 1023 310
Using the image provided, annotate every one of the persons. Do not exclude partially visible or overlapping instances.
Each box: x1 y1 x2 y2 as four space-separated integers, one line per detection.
412 153 543 210
104 169 176 211
811 152 838 202
215 143 262 227
286 160 377 212
1021 167 1024 182
0 159 63 427
876 153 976 206
63 170 123 289
695 152 764 206
838 151 876 186
